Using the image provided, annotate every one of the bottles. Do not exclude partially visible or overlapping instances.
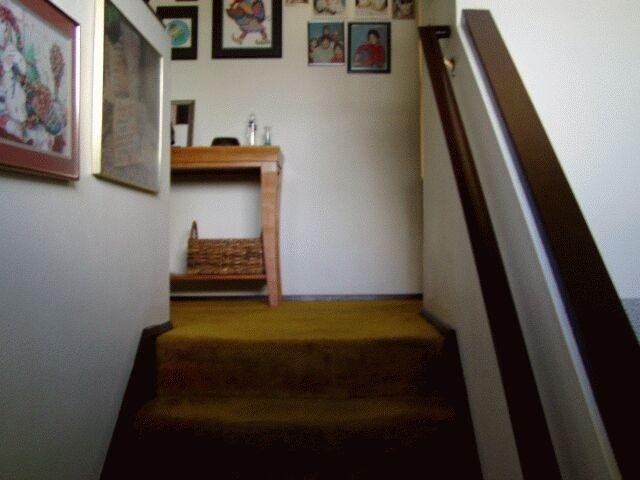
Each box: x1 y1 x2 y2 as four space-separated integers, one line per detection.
263 125 272 146
245 114 257 148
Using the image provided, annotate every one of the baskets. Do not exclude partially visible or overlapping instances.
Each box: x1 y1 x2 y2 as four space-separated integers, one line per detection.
186 220 265 275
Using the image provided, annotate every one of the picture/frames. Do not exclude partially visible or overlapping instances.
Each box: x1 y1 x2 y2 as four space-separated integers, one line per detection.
0 0 163 197
157 0 392 74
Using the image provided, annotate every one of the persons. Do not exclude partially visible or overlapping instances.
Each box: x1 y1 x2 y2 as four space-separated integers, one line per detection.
309 24 343 64
352 29 386 70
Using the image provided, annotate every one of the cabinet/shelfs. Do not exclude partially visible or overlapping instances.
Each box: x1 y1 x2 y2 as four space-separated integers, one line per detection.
170 145 280 306
